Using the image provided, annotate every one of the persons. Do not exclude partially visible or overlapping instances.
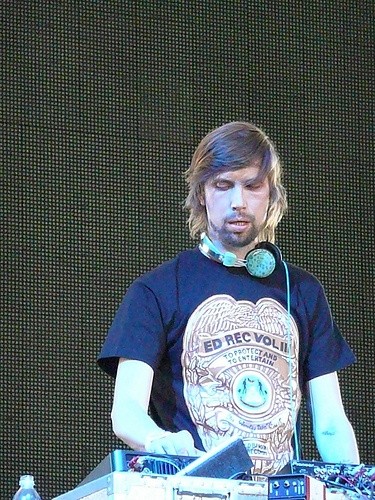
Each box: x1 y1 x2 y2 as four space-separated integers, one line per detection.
95 121 360 466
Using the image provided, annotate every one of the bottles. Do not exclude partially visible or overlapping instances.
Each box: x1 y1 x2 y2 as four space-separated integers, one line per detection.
12 475 42 500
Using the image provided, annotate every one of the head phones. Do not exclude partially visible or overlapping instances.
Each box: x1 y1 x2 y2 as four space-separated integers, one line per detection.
198 232 283 278
